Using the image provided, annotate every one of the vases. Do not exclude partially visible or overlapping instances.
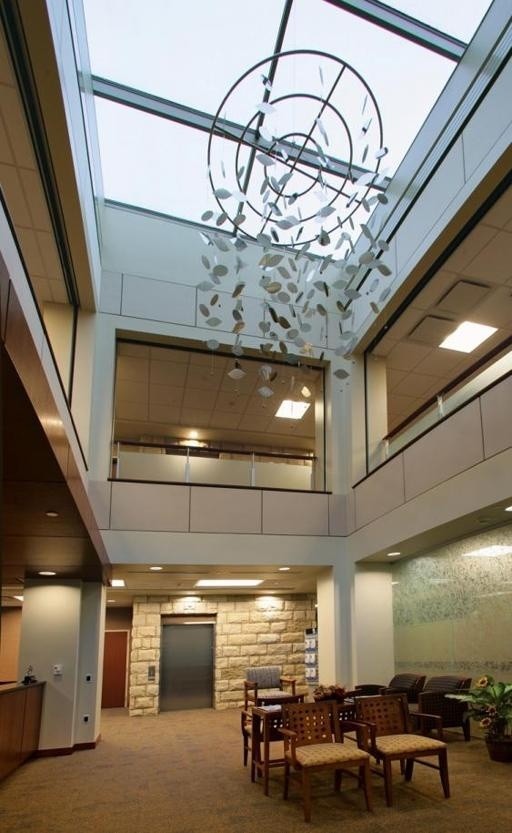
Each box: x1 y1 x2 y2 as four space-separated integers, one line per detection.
483 735 512 763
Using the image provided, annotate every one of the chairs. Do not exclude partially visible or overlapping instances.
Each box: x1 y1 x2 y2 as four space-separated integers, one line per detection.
239 663 472 823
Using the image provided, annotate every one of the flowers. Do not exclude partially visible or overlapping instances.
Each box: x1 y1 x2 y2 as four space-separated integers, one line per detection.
442 671 512 740
